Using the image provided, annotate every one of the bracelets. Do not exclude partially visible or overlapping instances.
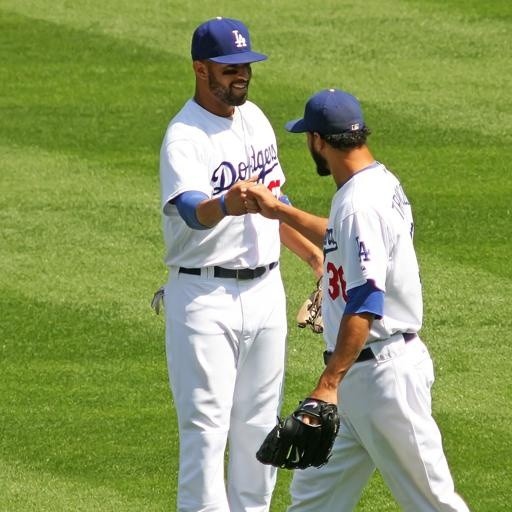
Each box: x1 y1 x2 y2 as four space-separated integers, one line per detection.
218 194 231 218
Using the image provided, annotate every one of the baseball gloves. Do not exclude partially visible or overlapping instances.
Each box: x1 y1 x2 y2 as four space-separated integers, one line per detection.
297 286 323 334
256 398 340 470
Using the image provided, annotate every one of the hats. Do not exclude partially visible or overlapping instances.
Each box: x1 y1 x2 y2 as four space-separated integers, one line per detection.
189 14 267 65
283 88 366 135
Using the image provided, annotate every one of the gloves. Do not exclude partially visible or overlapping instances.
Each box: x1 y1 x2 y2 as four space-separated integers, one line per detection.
148 286 166 316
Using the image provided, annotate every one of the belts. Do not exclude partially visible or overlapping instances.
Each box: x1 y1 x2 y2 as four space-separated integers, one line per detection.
323 331 417 366
177 263 281 280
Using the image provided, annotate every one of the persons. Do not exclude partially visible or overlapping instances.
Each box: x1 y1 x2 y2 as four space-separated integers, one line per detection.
158 15 327 512
243 84 472 512
